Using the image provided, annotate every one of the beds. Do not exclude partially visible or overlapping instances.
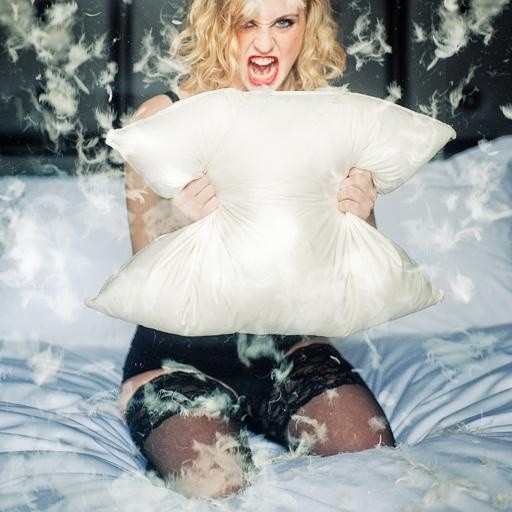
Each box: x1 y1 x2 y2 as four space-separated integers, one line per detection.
0 132 512 509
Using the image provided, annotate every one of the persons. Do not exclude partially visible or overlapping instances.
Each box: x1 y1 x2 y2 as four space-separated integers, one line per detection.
121 0 394 501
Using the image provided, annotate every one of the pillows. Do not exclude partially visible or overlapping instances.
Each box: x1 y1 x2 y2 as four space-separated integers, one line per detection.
0 174 139 346
372 136 511 335
83 87 457 337
